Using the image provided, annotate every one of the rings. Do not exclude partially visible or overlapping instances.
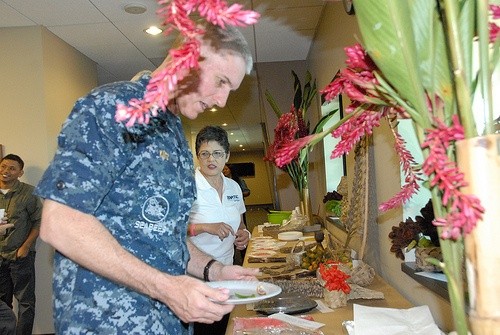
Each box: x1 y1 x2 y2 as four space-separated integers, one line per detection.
222 232 224 235
244 245 246 248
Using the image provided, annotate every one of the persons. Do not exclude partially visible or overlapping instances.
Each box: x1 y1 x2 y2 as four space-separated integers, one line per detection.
223 163 251 259
32 18 260 335
0 153 42 335
0 210 18 335
186 126 251 335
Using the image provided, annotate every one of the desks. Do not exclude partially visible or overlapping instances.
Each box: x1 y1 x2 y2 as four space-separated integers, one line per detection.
223 224 445 335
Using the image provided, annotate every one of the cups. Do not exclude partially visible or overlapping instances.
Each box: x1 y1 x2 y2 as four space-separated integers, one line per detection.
0 207 6 225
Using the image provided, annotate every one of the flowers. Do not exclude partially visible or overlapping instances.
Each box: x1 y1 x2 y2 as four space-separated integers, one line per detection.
115 0 500 335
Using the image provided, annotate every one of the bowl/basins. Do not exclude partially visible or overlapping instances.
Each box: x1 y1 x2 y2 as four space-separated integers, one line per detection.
276 230 306 241
267 213 292 224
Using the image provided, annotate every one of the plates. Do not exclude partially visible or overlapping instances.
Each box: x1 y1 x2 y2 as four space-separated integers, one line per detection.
248 234 288 260
199 280 283 307
253 293 321 317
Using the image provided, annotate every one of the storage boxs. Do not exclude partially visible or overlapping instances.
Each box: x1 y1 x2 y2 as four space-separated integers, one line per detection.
266 210 292 225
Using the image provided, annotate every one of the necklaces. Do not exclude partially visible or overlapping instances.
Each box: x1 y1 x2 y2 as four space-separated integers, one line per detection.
202 173 222 192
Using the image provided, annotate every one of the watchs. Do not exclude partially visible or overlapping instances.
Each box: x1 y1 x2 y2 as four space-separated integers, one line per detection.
243 228 251 239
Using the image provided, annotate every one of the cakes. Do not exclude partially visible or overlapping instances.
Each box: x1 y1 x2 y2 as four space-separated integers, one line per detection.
262 225 282 236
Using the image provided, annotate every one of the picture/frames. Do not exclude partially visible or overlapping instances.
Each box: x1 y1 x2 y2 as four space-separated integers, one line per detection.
299 189 314 227
454 133 500 335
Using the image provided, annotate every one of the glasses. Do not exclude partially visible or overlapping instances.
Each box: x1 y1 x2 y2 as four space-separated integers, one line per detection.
196 150 227 159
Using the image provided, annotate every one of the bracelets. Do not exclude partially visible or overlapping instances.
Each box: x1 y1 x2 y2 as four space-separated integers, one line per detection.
24 242 31 249
203 259 216 282
189 223 198 236
